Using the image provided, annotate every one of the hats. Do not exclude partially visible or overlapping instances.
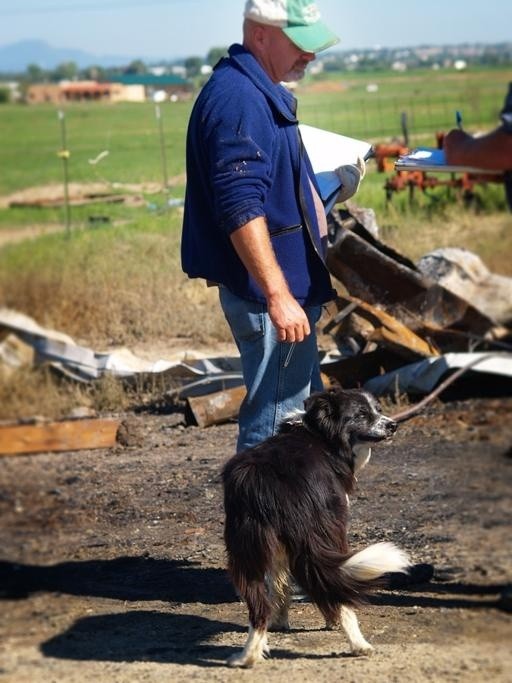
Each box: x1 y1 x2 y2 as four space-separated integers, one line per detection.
242 0 341 54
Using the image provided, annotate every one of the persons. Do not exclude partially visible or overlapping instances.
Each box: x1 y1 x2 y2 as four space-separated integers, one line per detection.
455 109 462 130
182 1 370 602
400 110 411 145
442 78 512 216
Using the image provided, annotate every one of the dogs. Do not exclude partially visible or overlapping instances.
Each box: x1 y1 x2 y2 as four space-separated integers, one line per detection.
221 384 412 670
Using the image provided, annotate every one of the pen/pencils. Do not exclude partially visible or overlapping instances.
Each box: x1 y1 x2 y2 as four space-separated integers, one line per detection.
282 343 296 370
456 111 463 129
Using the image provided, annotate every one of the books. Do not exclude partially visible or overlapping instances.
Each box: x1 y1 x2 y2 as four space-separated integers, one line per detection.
295 120 372 216
393 143 503 177
